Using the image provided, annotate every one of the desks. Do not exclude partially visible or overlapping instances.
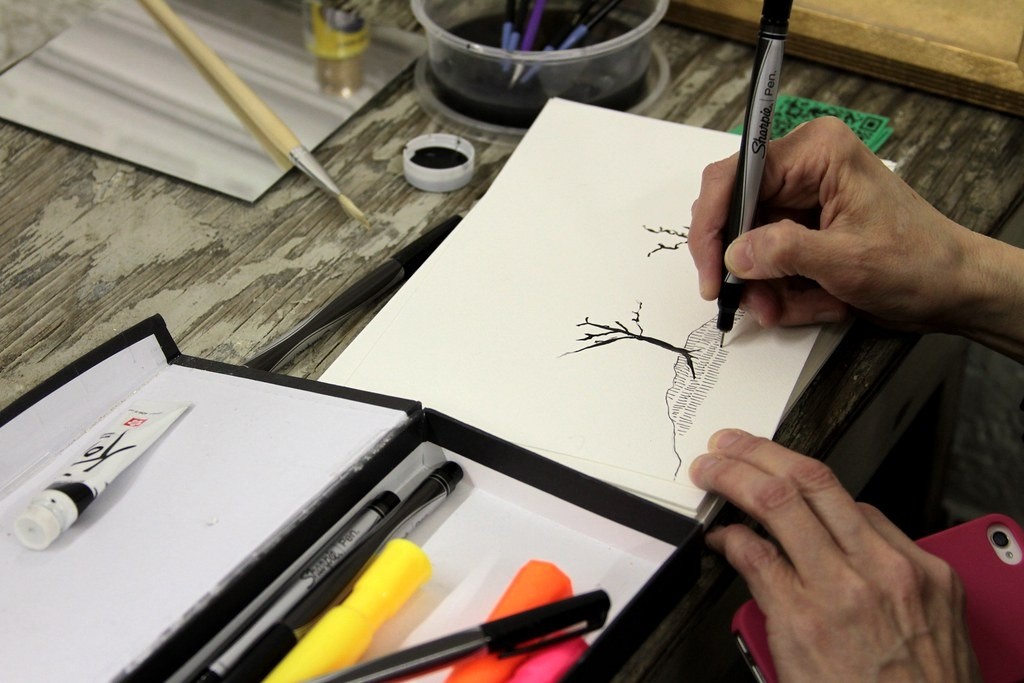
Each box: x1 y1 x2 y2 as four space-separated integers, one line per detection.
1 0 1024 683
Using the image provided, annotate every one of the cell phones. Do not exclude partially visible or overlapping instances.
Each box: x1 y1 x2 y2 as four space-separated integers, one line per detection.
733 514 1024 683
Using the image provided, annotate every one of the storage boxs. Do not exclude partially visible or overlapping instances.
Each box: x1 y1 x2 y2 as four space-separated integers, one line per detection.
1 313 705 683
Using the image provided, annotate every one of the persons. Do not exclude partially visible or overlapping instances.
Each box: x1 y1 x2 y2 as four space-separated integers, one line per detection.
687 114 1024 683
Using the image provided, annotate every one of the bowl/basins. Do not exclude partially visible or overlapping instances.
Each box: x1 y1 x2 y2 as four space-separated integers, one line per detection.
410 0 669 113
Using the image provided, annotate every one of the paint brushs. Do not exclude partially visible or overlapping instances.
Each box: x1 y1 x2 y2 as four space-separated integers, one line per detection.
501 0 619 91
143 0 372 229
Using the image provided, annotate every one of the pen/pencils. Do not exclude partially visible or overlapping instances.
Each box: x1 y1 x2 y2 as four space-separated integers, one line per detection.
718 1 793 349
188 458 617 683
241 213 465 373
